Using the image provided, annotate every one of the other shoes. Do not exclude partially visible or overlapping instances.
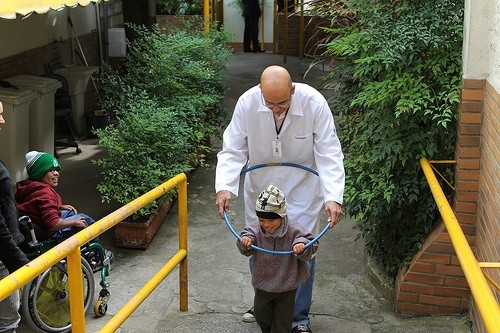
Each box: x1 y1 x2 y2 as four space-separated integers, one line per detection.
242 306 257 322
292 324 312 333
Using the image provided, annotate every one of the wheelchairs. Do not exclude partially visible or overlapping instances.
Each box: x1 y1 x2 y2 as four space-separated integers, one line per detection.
18 214 113 333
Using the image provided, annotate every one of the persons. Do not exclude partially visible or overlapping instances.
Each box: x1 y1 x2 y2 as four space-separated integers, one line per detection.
236 185 319 333
14 151 114 275
215 66 345 333
0 98 39 333
242 0 266 53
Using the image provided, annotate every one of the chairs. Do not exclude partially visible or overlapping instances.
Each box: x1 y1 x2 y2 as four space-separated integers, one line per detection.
44 74 83 158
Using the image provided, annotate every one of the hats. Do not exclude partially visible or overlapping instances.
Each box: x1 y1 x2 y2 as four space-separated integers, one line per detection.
25 151 61 181
255 185 288 219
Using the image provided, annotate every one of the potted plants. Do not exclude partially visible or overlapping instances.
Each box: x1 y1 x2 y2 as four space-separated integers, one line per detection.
156 0 204 28
90 13 237 250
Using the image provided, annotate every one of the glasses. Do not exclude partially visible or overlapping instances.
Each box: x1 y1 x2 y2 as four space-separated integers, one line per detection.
261 92 291 108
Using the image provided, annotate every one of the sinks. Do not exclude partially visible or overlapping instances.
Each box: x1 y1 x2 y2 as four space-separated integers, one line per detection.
54 64 98 96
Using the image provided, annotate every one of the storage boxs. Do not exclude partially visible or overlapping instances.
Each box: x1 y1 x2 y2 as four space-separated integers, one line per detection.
108 27 126 57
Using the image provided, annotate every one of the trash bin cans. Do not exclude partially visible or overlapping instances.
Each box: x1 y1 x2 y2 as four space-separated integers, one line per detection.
4 75 62 156
0 85 38 185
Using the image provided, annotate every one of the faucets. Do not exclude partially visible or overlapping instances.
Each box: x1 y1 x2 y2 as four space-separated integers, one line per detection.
55 35 65 45
